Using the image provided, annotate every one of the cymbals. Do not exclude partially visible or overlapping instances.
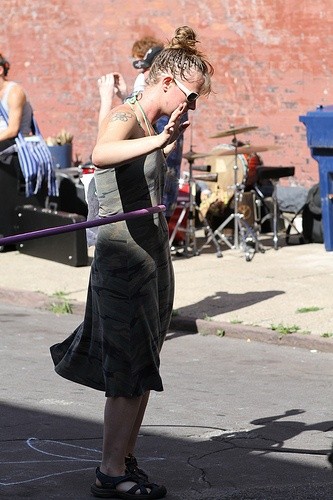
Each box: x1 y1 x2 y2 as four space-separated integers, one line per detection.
208 125 259 137
182 150 209 160
218 144 282 155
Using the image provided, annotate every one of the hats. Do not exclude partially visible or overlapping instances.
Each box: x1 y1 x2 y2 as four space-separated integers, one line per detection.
133 46 162 69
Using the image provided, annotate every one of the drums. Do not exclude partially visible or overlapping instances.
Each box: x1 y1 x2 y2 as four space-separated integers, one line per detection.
205 144 263 191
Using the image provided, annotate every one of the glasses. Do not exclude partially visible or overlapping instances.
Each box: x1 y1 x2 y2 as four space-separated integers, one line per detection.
161 74 200 103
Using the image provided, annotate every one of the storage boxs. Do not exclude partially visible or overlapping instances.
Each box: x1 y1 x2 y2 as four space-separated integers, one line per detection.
14 203 89 267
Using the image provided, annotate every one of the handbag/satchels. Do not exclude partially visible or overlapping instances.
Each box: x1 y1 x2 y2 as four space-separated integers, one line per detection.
15 135 59 197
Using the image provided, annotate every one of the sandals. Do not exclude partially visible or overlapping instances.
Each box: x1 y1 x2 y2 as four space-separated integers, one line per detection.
90 455 167 500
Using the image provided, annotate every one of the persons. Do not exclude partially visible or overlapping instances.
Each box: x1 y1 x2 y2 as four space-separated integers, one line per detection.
50 25 215 500
0 56 33 253
